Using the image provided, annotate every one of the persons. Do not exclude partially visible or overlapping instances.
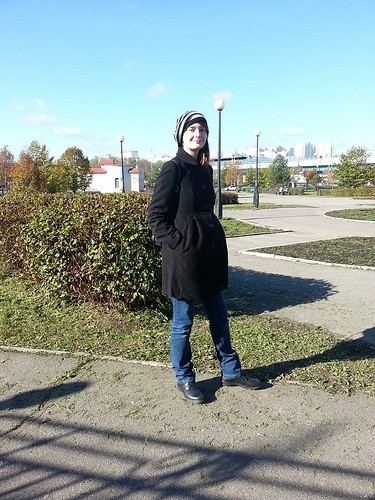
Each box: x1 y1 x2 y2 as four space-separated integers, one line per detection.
276 184 289 195
146 109 265 404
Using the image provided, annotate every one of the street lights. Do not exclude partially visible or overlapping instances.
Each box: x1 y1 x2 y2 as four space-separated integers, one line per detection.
119 135 125 193
253 128 261 209
214 98 223 220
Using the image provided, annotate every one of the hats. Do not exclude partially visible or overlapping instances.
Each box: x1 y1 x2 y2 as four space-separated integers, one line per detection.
175 111 208 151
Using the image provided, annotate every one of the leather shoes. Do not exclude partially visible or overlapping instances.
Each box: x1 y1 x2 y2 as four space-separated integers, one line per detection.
177 380 205 403
221 375 264 389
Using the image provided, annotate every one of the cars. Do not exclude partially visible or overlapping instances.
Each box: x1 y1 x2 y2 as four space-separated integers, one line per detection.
227 185 237 191
